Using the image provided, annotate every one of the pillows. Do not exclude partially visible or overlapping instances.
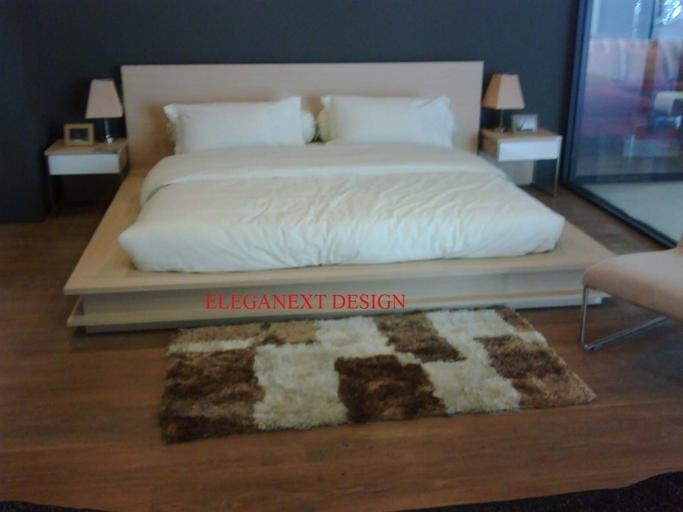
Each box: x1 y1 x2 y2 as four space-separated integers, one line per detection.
162 95 304 152
318 106 457 139
164 111 317 143
319 95 450 147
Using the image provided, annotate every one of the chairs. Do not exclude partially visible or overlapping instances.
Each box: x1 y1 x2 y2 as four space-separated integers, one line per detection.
579 233 683 353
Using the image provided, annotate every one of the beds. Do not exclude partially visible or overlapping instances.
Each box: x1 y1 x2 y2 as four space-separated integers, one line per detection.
62 61 615 331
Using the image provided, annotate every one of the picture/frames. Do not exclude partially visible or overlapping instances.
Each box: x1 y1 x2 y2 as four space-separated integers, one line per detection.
512 114 538 134
64 123 94 146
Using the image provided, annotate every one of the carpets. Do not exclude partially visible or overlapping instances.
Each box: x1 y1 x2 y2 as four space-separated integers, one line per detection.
157 305 597 446
640 327 683 380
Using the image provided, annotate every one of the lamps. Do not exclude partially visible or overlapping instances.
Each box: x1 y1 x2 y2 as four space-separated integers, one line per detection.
84 78 123 144
482 73 526 134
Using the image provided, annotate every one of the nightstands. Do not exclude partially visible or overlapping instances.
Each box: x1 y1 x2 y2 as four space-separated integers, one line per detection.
44 137 127 216
481 130 562 197
